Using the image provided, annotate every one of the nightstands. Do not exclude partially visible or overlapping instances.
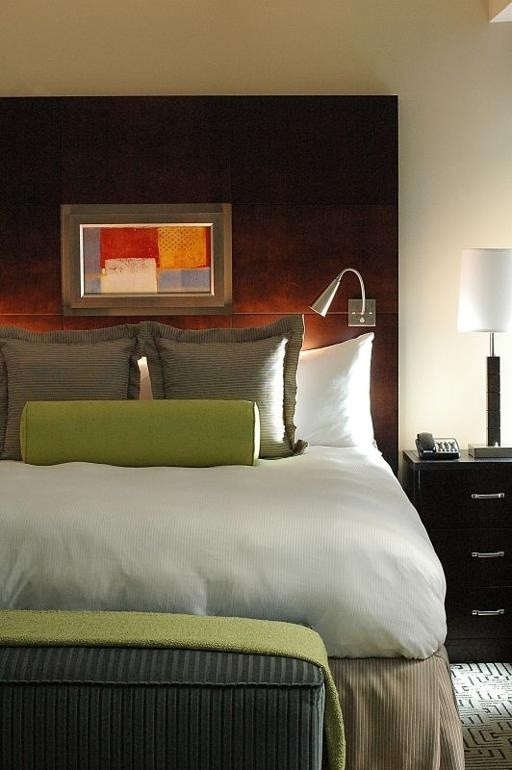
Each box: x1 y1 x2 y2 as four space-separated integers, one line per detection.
402 448 512 663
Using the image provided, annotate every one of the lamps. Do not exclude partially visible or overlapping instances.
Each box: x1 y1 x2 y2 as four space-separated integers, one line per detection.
456 247 512 458
311 269 377 328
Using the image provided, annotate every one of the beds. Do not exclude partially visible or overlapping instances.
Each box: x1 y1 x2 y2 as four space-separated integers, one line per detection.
0 442 467 770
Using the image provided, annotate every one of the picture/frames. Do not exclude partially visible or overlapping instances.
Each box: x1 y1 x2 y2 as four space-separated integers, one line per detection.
58 204 231 317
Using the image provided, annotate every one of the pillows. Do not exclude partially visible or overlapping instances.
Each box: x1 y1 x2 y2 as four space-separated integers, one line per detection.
135 331 374 447
19 399 265 466
139 316 309 461
1 320 141 466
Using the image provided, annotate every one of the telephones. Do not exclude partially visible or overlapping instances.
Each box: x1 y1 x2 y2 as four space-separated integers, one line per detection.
415 432 460 459
